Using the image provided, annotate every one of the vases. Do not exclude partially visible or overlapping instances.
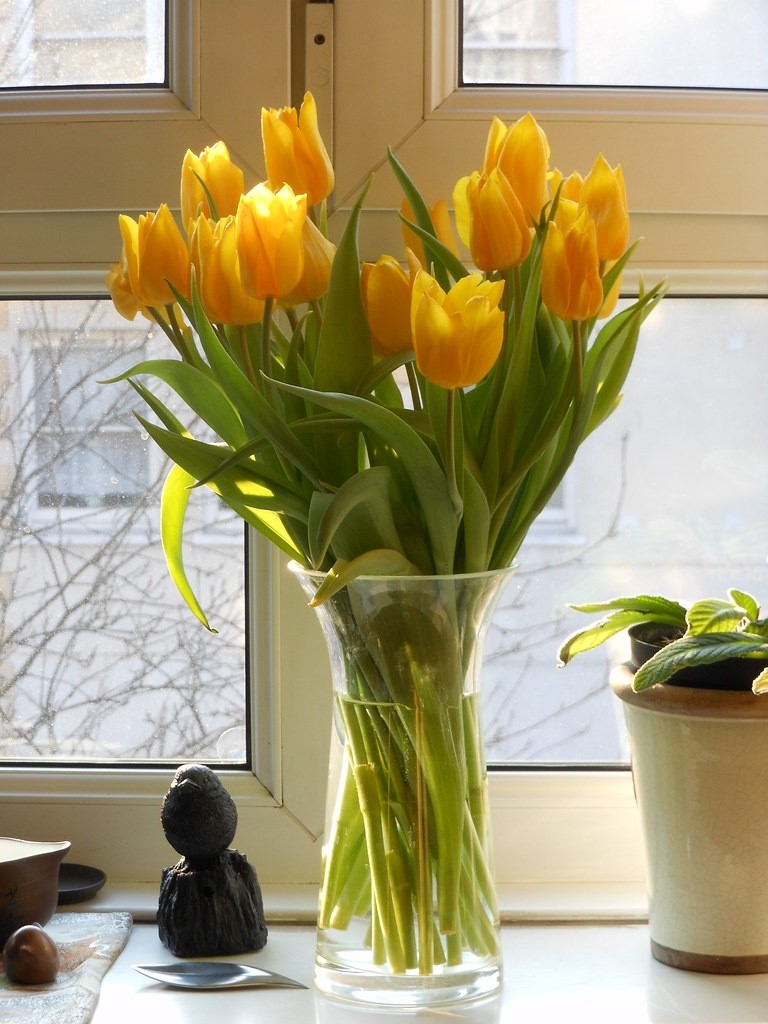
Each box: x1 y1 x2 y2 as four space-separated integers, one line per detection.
287 557 519 1010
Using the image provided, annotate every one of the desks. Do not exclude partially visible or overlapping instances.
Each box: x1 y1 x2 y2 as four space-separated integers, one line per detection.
82 915 768 1023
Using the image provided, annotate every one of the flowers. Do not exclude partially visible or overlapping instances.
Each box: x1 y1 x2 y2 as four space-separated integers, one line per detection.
95 89 667 974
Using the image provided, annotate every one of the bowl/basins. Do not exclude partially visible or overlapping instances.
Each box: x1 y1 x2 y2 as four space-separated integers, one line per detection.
0 835 71 947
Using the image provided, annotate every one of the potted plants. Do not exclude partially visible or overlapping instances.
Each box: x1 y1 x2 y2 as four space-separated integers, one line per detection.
557 588 768 975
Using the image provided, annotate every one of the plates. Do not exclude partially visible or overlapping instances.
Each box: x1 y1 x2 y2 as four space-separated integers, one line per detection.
57 861 107 907
